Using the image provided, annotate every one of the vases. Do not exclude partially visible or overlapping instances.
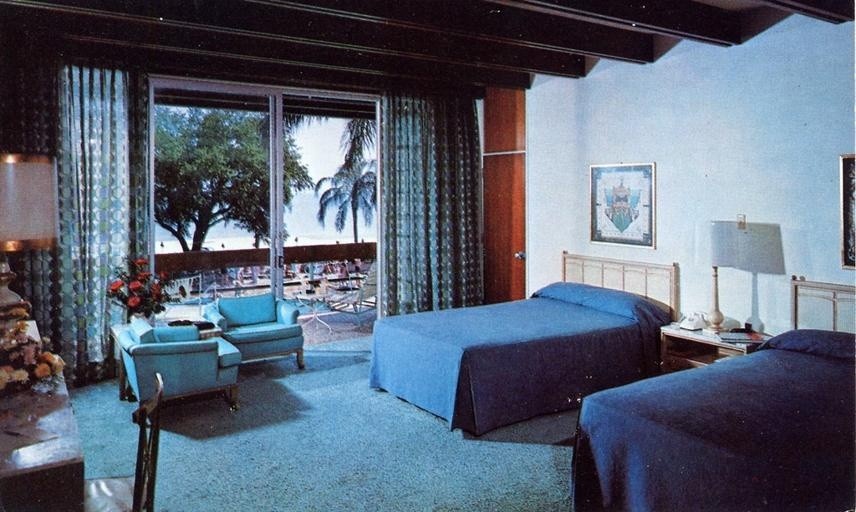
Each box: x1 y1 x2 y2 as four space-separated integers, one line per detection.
130 311 156 328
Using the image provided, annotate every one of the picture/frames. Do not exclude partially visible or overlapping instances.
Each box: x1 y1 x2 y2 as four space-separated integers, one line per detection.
839 154 856 270
589 162 655 249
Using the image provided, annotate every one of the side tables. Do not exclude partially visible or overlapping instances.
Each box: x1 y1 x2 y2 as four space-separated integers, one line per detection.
162 319 223 339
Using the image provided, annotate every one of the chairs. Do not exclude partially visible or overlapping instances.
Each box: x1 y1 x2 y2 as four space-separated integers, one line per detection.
202 297 305 368
226 259 378 330
82 372 165 512
110 324 241 425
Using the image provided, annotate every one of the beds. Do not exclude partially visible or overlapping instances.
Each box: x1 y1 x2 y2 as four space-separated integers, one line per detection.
375 249 679 437
582 276 856 512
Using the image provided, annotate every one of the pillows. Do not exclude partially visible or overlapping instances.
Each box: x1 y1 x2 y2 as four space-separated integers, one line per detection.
217 293 276 327
129 316 160 343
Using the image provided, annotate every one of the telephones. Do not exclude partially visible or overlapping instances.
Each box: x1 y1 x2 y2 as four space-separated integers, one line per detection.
678 311 712 330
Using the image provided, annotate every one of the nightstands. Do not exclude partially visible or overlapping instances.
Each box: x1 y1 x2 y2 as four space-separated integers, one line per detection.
660 324 773 375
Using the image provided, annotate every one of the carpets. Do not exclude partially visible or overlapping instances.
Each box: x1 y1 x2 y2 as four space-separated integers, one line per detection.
66 338 582 512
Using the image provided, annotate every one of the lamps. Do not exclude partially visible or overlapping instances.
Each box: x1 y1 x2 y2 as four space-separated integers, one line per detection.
0 154 61 307
692 221 739 338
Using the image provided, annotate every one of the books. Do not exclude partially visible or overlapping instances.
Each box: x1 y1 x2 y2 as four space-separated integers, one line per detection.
715 331 775 346
684 347 744 368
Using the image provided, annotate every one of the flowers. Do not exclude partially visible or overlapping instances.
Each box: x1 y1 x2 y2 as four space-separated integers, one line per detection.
0 307 66 424
103 257 186 317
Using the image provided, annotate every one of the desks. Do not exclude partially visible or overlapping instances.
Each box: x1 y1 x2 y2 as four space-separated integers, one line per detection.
292 295 337 336
0 321 85 512
